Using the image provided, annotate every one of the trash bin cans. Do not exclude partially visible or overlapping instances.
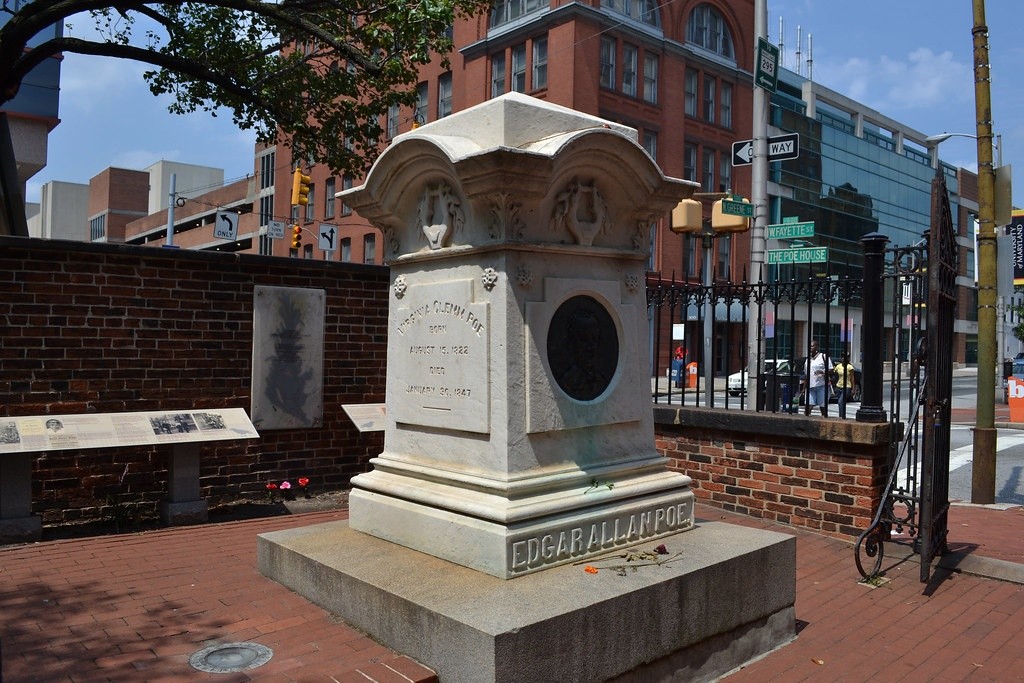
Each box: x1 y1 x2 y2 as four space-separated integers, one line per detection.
765 372 800 415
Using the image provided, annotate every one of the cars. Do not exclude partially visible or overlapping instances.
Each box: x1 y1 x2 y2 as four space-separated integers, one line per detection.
727 357 861 404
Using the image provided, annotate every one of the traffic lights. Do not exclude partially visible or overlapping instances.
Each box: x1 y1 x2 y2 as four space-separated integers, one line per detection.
291 225 302 250
290 172 311 207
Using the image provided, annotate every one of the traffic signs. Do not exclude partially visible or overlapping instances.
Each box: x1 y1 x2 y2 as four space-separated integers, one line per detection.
721 199 753 218
766 247 829 264
766 221 816 239
268 220 285 239
731 133 800 167
754 36 780 95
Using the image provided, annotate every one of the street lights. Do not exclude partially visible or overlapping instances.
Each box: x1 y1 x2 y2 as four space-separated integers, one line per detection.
923 132 1005 389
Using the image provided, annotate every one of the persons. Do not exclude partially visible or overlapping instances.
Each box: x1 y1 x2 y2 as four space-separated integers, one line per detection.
800 340 834 418
675 342 689 360
832 351 855 418
